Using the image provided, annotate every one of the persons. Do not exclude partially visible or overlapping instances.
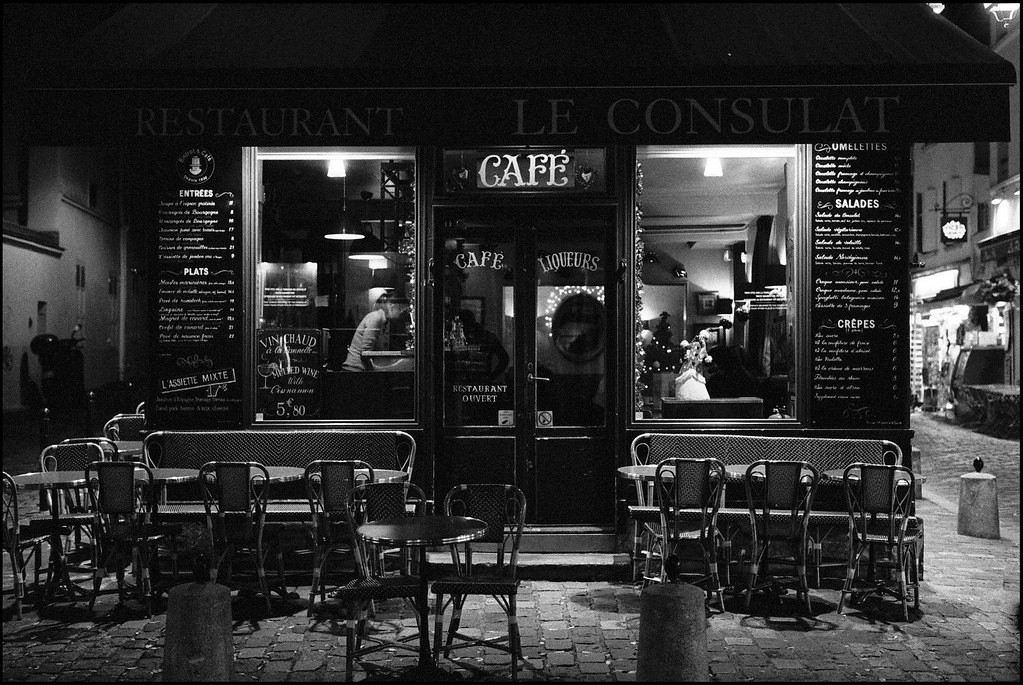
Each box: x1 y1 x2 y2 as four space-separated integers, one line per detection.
460 309 510 381
342 292 408 372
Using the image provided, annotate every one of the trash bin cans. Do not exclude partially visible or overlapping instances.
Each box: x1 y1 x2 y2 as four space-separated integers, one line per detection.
951 345 1006 426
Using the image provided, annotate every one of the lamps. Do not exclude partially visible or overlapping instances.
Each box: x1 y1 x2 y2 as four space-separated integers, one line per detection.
349 228 384 258
324 162 365 242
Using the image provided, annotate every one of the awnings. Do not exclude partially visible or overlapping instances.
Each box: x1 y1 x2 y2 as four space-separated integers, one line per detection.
18 0 1014 145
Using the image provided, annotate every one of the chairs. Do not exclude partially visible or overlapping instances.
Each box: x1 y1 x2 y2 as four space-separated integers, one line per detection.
835 462 923 620
39 402 145 569
304 458 383 617
426 483 527 685
197 459 286 614
743 459 820 617
345 482 432 684
656 456 727 612
3 471 65 619
84 460 155 616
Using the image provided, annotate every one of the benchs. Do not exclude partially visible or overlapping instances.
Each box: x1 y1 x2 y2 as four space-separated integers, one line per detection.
631 431 901 581
139 430 413 577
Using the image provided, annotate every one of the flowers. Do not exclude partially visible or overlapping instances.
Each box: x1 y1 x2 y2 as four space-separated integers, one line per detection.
680 339 714 367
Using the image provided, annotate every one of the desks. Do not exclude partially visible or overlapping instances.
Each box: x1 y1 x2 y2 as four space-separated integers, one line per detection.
821 467 927 600
3 471 99 602
308 470 408 591
202 466 307 597
617 464 717 588
965 385 1019 437
355 513 489 684
720 466 821 580
99 441 143 461
134 467 199 604
